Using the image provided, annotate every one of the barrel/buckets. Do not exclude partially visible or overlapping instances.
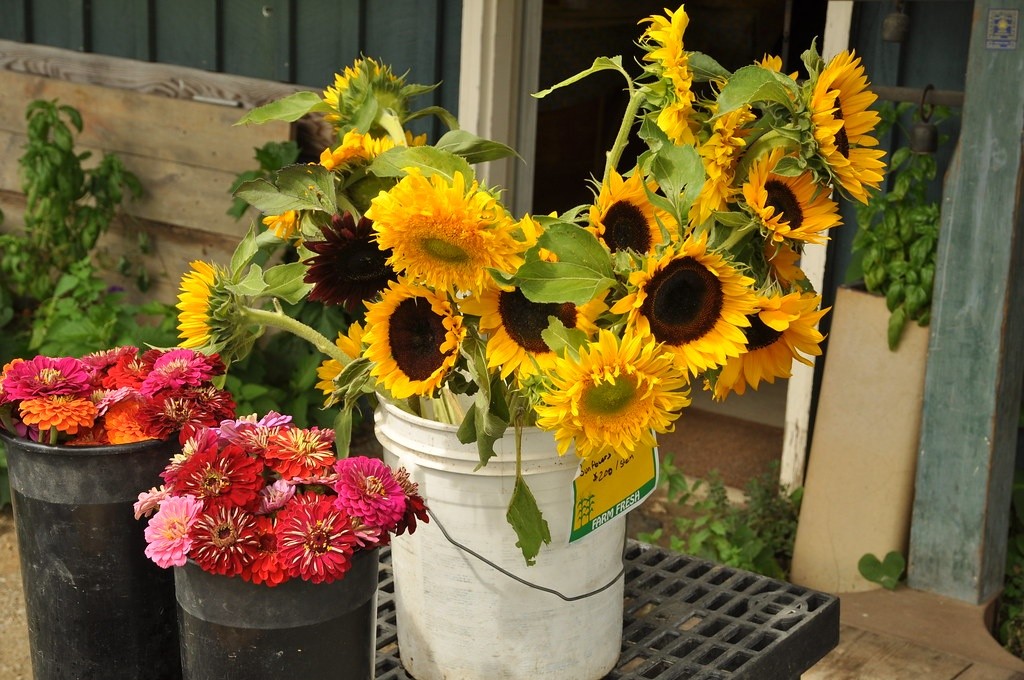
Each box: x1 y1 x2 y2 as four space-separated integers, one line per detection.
372 372 628 680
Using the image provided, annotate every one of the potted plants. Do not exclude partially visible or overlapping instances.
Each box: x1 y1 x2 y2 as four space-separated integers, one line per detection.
788 102 961 594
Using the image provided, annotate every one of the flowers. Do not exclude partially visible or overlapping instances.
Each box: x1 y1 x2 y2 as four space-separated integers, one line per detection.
133 409 430 589
0 345 238 447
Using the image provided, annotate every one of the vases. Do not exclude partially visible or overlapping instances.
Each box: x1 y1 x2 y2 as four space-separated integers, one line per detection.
173 548 379 680
0 434 183 680
